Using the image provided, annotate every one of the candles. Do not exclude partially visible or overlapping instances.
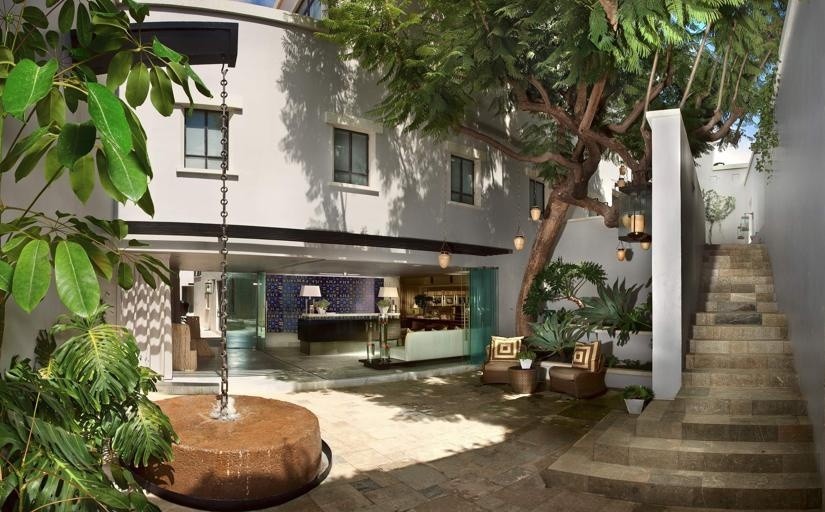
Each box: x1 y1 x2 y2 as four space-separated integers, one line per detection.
632 215 645 233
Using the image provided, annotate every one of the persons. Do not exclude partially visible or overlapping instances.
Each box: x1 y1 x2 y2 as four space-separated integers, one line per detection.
178 301 192 325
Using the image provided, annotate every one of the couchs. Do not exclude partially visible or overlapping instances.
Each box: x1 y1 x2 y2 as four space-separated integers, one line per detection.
388 326 495 366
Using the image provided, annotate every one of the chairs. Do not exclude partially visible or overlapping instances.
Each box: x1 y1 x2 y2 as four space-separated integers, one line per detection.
481 336 608 401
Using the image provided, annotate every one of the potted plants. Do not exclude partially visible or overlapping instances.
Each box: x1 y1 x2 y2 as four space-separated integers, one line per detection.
375 300 392 313
313 298 331 314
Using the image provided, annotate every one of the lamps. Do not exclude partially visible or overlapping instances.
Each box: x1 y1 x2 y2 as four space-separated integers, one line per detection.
377 287 400 312
438 150 452 269
512 154 543 251
299 285 322 313
616 240 626 261
